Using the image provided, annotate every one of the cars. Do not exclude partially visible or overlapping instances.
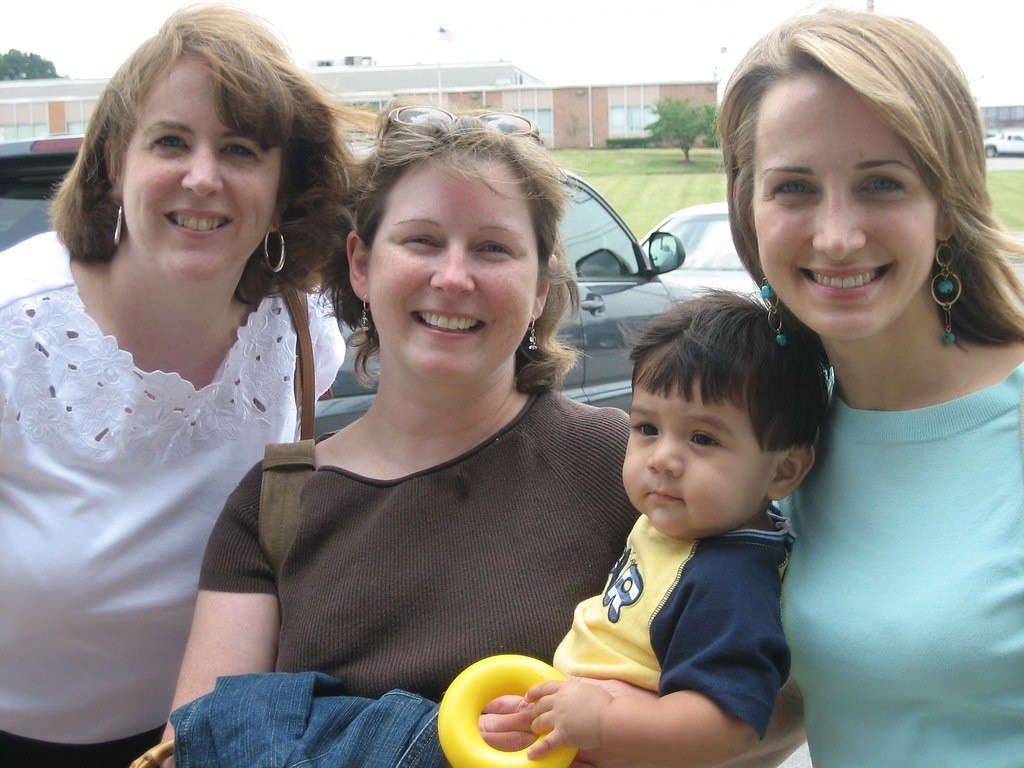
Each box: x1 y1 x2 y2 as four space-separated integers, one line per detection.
0 131 688 445
982 131 1024 157
638 199 837 391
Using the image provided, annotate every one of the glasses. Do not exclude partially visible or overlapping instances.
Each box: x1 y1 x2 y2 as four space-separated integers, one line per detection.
372 106 544 175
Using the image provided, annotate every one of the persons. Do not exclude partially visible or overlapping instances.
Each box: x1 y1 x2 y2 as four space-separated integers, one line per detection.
160 97 644 768
524 283 831 768
0 4 359 768
719 10 1023 768
